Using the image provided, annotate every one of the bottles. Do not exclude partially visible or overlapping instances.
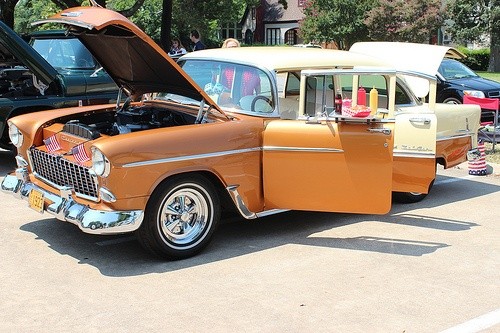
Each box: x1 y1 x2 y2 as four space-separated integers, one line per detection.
357 85 367 106
334 94 342 116
370 84 378 116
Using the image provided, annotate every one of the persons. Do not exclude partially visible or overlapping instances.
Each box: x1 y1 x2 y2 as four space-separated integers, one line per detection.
211 37 262 98
190 29 205 52
168 38 187 61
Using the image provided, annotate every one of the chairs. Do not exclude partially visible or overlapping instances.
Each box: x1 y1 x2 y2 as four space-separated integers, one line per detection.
463 95 499 153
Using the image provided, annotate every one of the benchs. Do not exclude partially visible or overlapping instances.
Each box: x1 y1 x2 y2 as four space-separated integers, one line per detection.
240 95 322 118
303 88 388 110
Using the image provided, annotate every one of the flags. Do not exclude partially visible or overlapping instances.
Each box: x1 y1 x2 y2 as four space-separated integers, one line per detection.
71 143 90 165
43 135 62 153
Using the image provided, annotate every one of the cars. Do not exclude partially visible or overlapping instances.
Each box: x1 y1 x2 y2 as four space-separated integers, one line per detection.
1 6 480 259
434 58 500 119
0 20 126 149
